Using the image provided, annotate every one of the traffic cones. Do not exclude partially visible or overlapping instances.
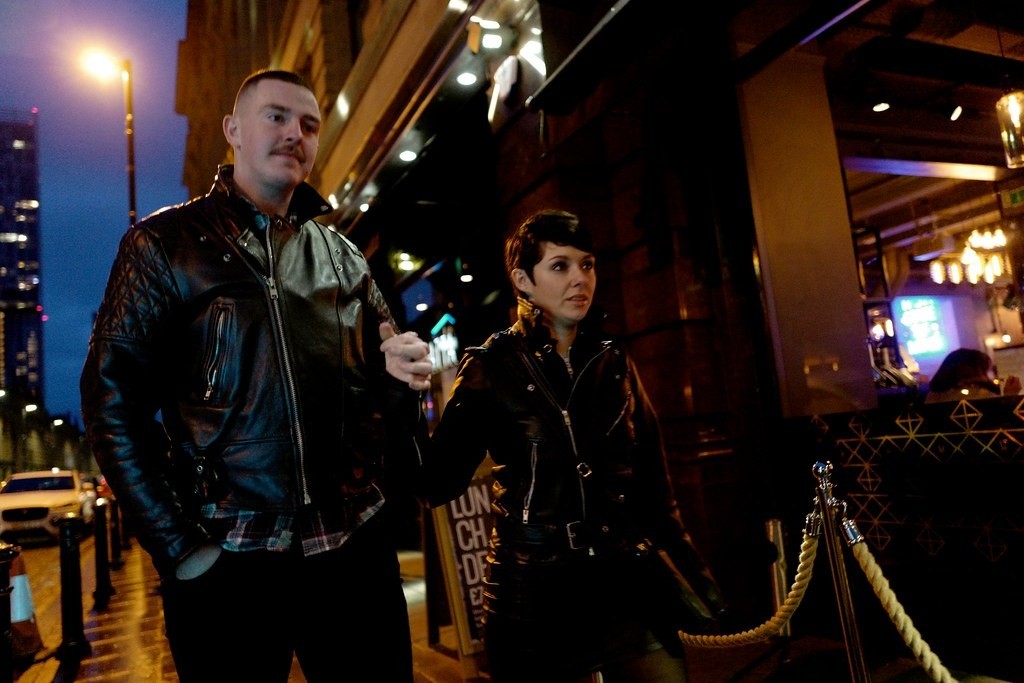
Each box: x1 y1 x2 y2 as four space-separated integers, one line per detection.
9 549 45 657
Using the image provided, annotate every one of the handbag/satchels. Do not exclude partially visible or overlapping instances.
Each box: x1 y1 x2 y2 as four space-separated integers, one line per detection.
633 539 713 657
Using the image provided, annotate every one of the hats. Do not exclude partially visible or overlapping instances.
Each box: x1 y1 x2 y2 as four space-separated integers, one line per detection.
930 348 1000 394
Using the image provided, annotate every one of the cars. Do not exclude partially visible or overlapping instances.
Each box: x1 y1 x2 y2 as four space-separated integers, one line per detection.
1 467 98 541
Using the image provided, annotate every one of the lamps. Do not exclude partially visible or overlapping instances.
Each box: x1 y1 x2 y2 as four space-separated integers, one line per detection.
995 18 1024 170
911 181 1006 285
457 55 478 85
397 132 421 161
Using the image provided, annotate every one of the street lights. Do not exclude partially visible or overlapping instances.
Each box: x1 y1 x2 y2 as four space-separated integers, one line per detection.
81 49 137 224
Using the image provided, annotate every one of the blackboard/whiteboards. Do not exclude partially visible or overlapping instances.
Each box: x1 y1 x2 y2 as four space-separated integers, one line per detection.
421 470 497 655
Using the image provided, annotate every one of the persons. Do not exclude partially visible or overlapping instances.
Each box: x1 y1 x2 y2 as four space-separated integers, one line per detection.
924 348 1023 405
80 69 436 683
427 210 726 683
